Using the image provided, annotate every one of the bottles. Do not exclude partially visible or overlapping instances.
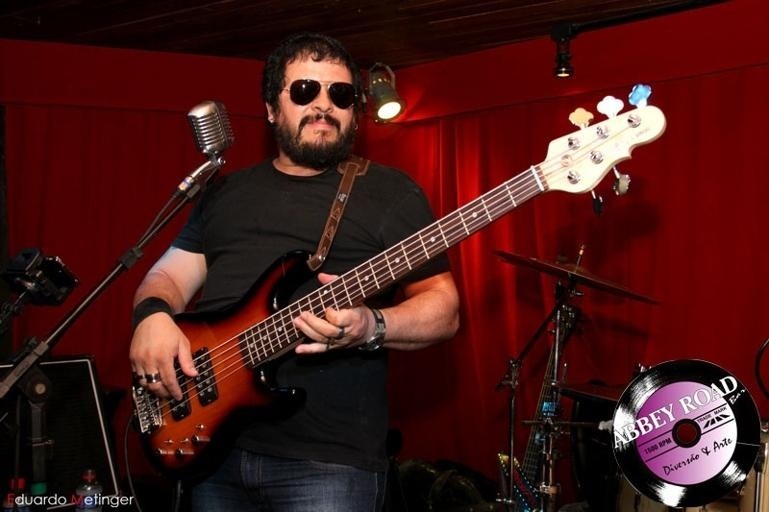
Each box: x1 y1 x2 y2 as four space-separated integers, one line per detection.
4 476 49 512
73 466 105 512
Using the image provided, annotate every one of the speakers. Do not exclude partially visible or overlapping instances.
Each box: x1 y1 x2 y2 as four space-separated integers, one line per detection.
0 353 123 512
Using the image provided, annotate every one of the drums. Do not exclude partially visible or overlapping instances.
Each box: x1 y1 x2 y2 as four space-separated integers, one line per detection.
620 418 768 512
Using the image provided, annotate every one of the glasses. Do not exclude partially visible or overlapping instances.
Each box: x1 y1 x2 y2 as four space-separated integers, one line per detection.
278 77 357 111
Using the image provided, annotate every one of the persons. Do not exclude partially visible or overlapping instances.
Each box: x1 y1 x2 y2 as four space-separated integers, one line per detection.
128 33 462 510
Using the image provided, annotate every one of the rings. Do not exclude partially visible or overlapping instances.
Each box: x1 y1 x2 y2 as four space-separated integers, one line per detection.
335 325 346 339
132 371 137 378
325 336 334 345
138 374 146 380
327 343 330 351
145 373 160 383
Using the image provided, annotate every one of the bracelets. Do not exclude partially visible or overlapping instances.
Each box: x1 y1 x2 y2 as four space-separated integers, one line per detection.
134 296 170 328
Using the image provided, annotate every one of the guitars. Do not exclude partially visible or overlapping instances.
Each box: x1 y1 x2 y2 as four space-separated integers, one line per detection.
110 80 667 488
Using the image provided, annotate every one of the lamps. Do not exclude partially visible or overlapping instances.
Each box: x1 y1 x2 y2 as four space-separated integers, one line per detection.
359 63 407 123
554 26 573 79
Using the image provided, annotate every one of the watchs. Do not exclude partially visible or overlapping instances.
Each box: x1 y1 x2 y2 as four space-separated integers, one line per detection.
359 308 386 353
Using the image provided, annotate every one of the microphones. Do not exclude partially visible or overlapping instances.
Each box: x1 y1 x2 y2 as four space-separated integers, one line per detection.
184 99 235 174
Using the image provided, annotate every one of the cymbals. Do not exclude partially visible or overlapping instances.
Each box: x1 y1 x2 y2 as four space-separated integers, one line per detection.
555 381 621 405
495 250 662 306
